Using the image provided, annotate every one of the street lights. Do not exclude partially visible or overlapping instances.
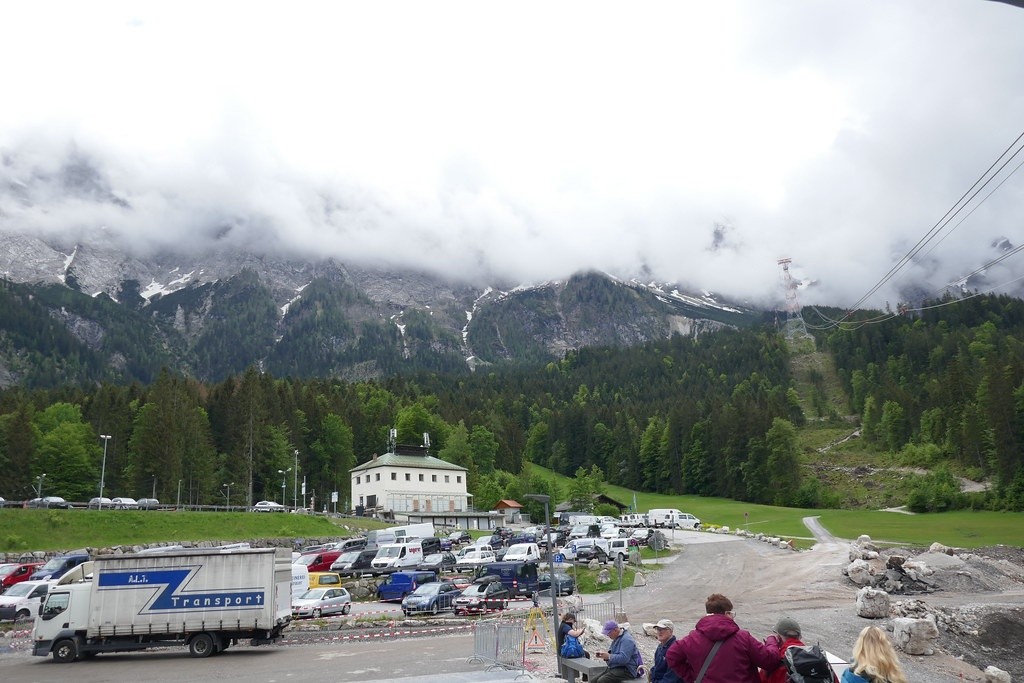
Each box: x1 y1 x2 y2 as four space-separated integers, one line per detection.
278 468 292 504
97 434 113 511
223 482 234 512
522 494 564 678
177 479 182 510
152 474 156 498
294 449 298 513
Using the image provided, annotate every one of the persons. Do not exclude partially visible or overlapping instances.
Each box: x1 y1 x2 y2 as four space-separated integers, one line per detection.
841 626 907 683
760 618 839 683
556 613 590 682
666 593 779 683
587 619 644 683
648 619 684 683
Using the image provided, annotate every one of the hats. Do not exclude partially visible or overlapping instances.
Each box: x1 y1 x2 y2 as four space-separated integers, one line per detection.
771 617 801 638
601 621 618 635
653 619 674 631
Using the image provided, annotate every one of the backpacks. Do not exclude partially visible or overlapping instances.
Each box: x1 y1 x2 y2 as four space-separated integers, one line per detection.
561 635 585 659
781 646 834 683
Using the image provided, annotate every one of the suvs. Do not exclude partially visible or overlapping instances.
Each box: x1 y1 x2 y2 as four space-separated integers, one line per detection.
451 575 511 615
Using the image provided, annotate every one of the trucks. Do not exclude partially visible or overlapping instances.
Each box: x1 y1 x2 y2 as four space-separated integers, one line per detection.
31 547 294 663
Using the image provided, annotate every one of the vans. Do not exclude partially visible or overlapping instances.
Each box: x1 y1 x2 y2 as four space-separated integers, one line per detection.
365 508 701 599
308 572 342 590
292 537 369 565
292 550 343 573
369 542 424 579
0 554 89 623
291 564 311 599
328 550 379 580
376 571 437 601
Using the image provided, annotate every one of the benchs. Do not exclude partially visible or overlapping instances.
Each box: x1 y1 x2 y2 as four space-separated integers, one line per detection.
621 676 649 683
560 657 608 683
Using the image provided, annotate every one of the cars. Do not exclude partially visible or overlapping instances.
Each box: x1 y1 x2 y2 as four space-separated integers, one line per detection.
416 552 457 575
112 497 139 510
401 581 461 616
22 496 68 510
252 501 285 512
88 497 115 510
292 588 352 619
136 498 160 510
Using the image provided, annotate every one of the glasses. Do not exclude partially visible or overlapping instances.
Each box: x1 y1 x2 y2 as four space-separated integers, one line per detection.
726 611 736 618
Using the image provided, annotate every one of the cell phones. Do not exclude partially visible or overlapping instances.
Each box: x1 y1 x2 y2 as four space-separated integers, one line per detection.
597 653 602 656
